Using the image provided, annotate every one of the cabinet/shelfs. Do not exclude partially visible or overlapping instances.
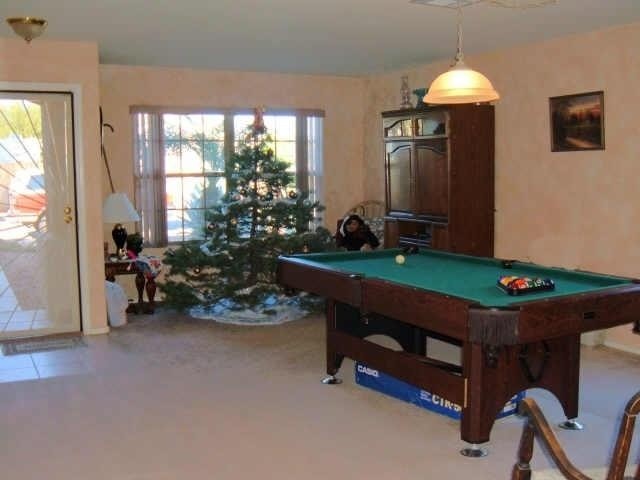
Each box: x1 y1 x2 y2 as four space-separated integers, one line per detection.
380 105 496 257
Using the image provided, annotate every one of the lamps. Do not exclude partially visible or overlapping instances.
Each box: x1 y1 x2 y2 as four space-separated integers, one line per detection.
102 192 141 259
5 16 48 44
422 0 501 105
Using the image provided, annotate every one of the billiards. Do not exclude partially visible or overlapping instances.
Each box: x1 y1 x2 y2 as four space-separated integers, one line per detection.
395 255 405 264
497 275 553 288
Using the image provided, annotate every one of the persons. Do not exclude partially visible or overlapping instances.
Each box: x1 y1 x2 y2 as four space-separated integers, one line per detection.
337 214 380 254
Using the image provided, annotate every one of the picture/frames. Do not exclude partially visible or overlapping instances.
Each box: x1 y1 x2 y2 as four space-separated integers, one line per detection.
548 89 606 153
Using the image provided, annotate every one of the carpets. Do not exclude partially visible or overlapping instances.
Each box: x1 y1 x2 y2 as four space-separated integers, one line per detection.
1 332 87 356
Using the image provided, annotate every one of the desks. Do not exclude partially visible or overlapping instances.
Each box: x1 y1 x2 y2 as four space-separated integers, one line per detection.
276 248 640 459
105 254 161 314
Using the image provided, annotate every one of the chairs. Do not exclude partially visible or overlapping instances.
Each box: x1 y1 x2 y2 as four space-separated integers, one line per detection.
339 199 385 250
512 392 640 480
